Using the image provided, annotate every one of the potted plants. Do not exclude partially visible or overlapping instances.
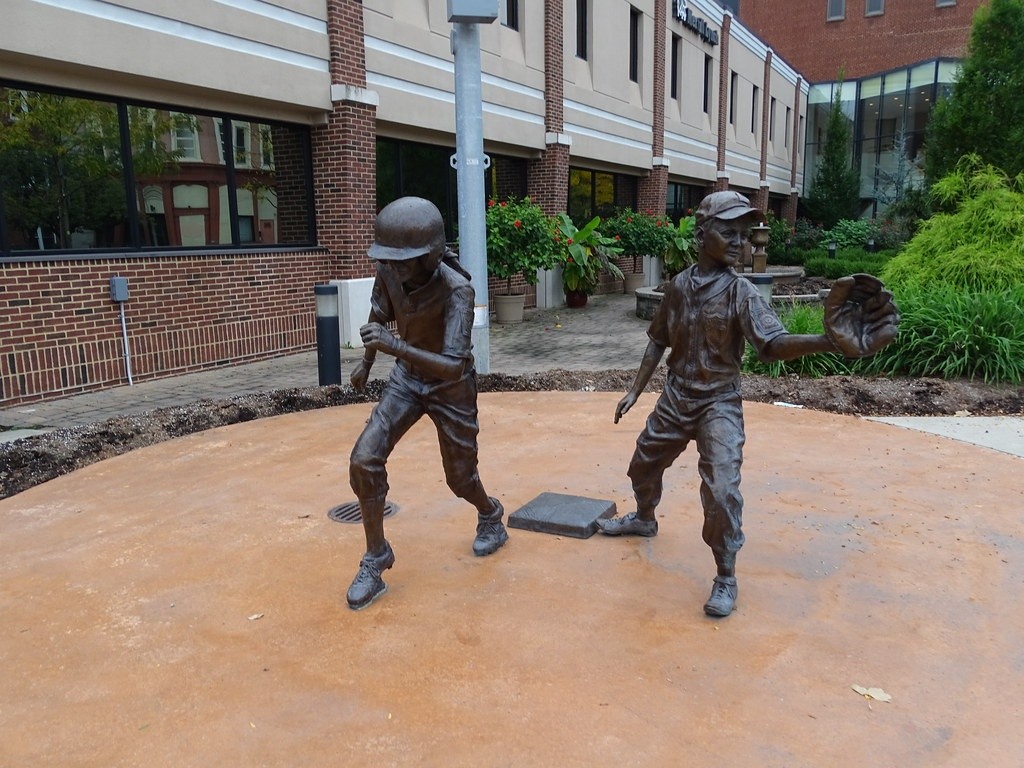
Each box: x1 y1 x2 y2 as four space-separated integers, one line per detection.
634 216 704 322
547 213 625 306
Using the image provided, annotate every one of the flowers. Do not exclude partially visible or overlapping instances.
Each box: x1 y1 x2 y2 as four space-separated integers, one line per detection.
453 193 574 294
596 207 676 273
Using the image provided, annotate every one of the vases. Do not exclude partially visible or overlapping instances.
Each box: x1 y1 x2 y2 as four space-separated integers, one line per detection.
624 274 646 295
494 292 525 323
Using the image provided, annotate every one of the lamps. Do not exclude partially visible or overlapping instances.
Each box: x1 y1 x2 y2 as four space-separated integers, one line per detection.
868 238 874 246
828 240 837 251
314 284 339 317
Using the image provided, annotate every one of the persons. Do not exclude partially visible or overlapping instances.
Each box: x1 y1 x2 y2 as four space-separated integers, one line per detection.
344 196 509 611
596 190 903 617
